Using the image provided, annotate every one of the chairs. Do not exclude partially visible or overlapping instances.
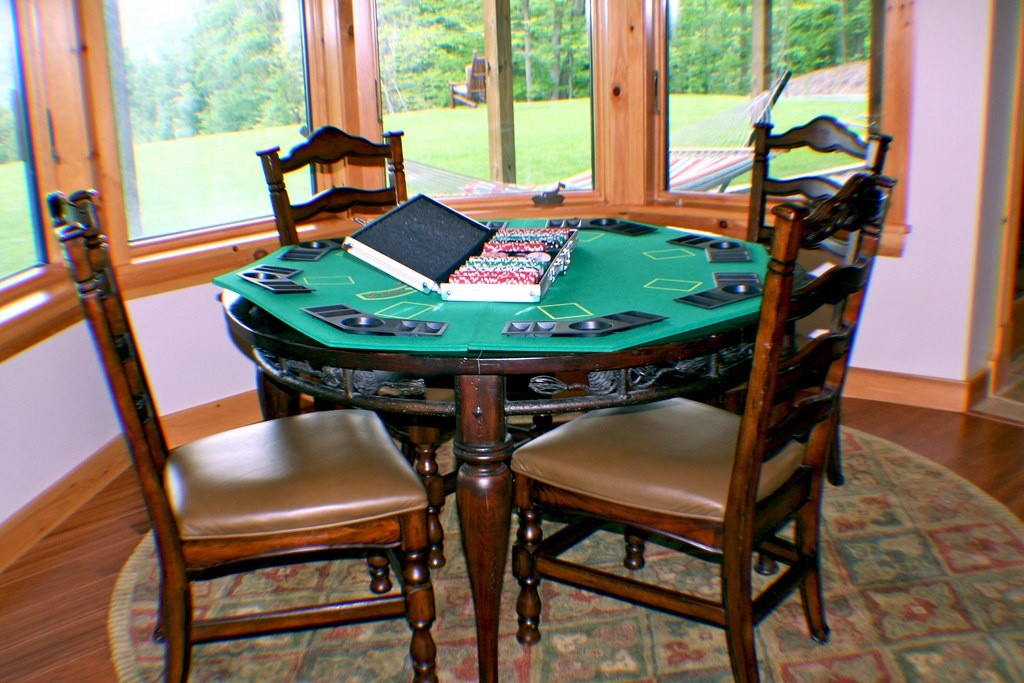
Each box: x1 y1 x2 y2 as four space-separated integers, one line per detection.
450 50 486 108
45 114 898 683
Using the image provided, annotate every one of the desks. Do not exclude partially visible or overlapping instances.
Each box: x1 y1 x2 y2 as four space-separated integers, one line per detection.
219 216 811 683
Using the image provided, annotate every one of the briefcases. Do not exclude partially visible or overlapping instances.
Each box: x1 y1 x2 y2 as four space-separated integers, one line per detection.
342 192 578 302
